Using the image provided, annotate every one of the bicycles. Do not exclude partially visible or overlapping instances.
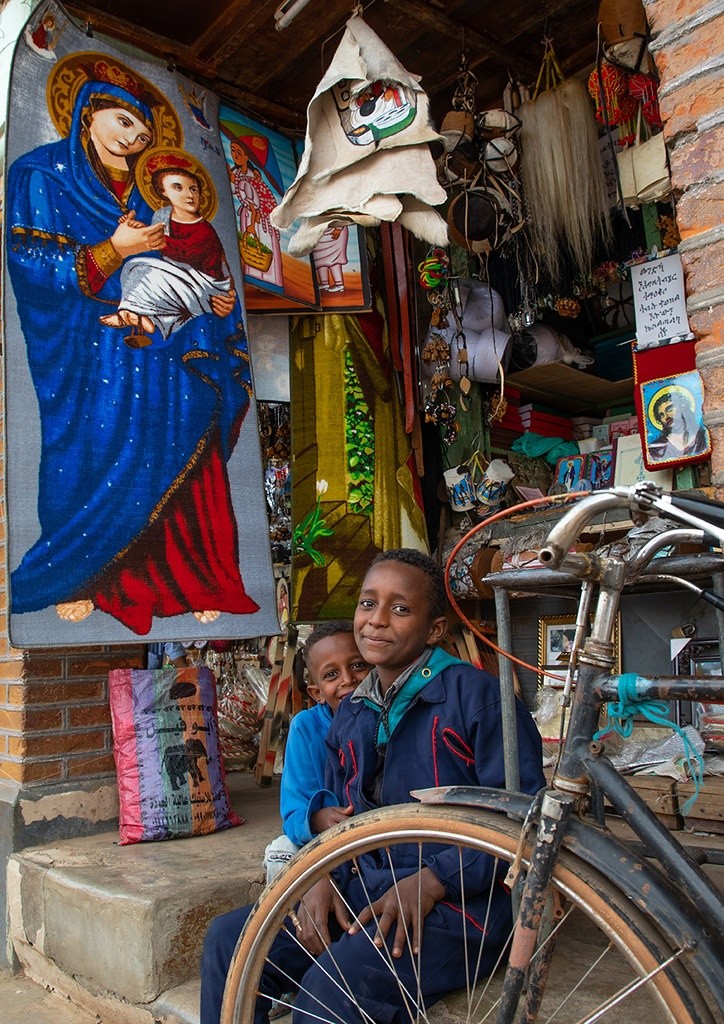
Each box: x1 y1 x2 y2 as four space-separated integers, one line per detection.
222 480 724 1024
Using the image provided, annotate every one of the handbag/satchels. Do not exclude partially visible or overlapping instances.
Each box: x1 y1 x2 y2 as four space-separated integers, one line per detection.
107 666 249 847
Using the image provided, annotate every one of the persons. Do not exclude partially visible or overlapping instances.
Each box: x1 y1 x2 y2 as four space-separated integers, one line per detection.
597 429 605 441
146 641 189 670
198 548 548 1024
